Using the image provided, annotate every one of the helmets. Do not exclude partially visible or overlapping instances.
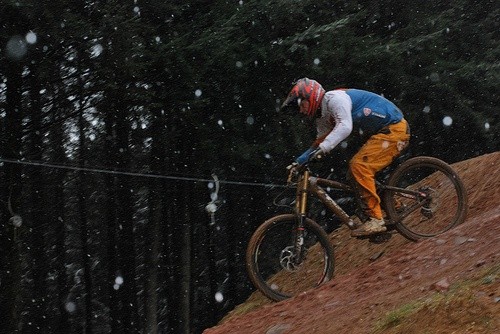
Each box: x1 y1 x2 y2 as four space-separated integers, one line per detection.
281 77 326 120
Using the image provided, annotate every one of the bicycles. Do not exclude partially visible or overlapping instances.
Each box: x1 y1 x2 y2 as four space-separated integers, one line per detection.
245 151 469 304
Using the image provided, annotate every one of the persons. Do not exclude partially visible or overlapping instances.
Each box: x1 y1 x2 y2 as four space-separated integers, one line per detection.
280 78 410 242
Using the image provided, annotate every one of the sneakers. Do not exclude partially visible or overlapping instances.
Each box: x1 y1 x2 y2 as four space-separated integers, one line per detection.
351 217 387 236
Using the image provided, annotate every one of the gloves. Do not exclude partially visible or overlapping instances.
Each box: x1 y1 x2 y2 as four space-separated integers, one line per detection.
296 146 317 164
308 146 324 161
